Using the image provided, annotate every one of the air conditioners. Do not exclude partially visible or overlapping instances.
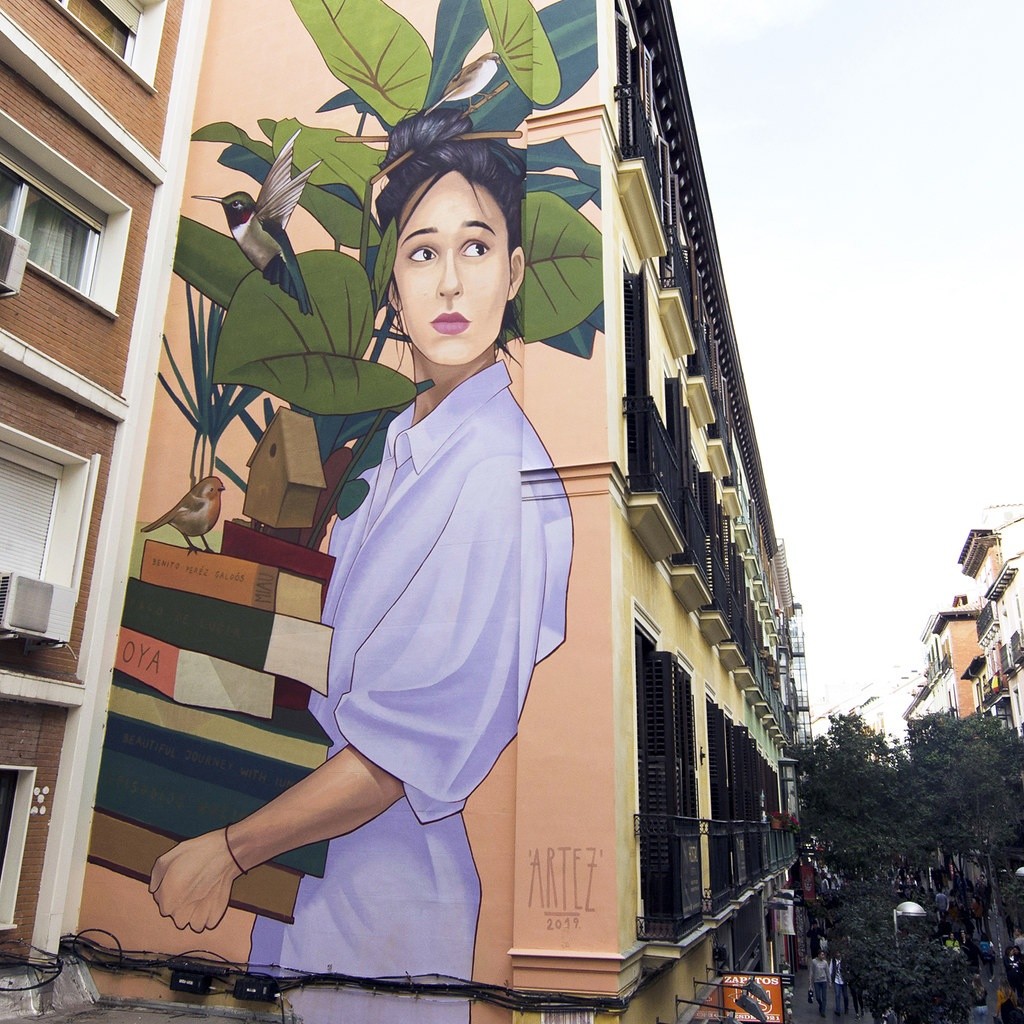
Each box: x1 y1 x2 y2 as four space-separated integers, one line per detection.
0 225 32 293
0 571 77 644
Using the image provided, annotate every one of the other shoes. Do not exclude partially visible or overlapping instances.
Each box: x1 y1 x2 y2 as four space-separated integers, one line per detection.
854 1015 860 1020
834 1011 841 1016
821 1013 825 1018
844 1008 849 1014
819 1006 821 1012
861 1009 864 1017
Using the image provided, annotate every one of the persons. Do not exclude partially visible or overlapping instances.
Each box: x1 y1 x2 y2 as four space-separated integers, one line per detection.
1003 913 1024 999
970 973 988 1024
829 949 849 1016
1009 997 1024 1024
808 950 832 1018
978 933 995 982
992 973 1017 1024
806 860 989 964
849 982 865 1021
881 1007 909 1024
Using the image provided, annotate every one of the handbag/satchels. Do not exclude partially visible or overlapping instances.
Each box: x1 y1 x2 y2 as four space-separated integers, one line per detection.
808 987 814 1004
993 1015 1002 1024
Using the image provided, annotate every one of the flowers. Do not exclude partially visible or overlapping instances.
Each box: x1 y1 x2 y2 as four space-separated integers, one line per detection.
783 811 801 834
769 811 786 822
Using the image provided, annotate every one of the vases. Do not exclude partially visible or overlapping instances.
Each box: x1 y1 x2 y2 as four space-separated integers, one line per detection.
772 821 783 830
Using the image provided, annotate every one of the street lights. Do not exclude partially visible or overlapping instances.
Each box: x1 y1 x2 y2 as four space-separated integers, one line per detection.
893 898 926 952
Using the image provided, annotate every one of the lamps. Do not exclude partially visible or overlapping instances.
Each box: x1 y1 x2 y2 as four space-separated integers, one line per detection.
675 994 743 1024
763 901 788 910
693 976 767 1023
773 892 793 900
705 964 772 1005
